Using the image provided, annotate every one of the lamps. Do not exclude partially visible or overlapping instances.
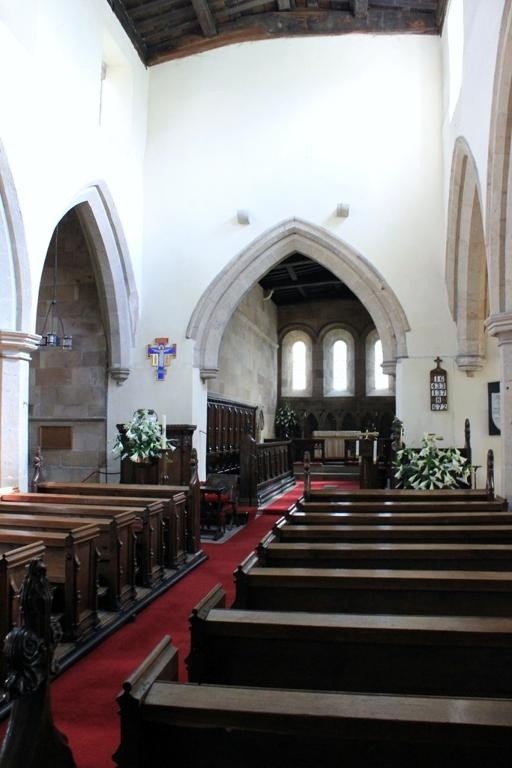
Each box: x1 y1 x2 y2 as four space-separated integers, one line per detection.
37 224 74 352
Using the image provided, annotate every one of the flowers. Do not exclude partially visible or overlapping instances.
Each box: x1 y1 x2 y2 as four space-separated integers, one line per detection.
107 408 178 466
390 431 471 491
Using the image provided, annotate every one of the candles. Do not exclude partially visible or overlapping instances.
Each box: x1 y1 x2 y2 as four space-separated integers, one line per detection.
161 415 166 449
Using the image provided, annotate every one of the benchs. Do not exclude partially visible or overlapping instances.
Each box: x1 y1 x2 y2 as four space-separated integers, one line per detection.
110 634 512 767
295 494 508 512
284 503 512 524
0 482 190 649
272 516 512 536
183 582 512 688
228 548 512 612
254 530 512 559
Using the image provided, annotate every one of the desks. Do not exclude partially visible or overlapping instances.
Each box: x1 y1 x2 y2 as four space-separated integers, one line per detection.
263 437 326 466
311 429 363 458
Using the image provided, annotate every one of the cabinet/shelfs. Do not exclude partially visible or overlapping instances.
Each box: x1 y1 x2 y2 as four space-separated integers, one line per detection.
236 431 297 508
207 392 259 475
